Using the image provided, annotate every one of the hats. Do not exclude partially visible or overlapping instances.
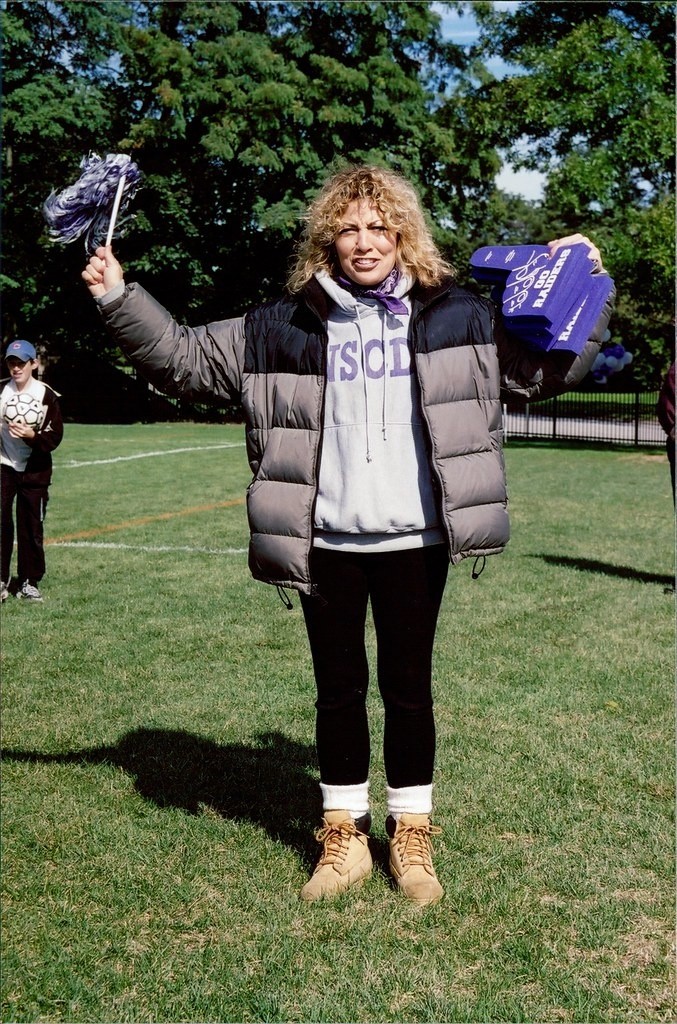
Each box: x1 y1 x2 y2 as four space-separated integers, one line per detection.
5 340 36 361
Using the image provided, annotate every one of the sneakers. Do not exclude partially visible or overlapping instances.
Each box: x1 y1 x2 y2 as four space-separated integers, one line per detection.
385 813 443 905
0 576 12 603
301 809 373 901
16 579 44 603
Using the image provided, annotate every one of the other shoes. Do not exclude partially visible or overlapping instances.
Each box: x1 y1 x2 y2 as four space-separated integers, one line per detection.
664 587 675 596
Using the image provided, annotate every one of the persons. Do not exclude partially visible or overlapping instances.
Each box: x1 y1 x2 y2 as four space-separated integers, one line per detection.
81 167 614 903
0 340 64 601
